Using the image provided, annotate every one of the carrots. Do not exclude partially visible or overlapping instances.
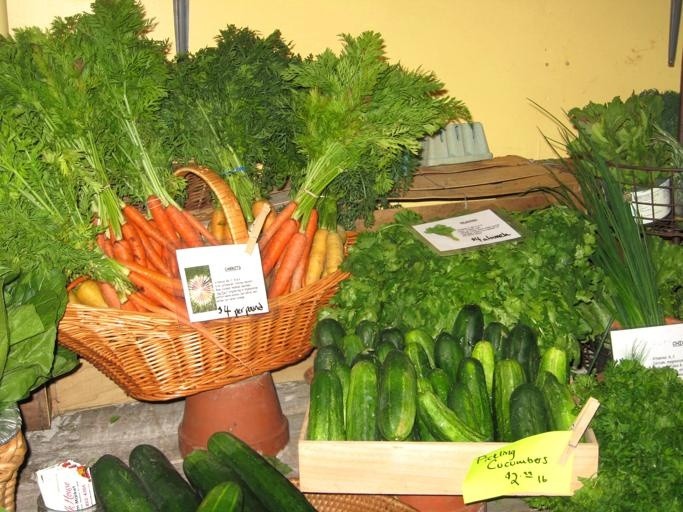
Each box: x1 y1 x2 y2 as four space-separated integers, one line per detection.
68 195 346 388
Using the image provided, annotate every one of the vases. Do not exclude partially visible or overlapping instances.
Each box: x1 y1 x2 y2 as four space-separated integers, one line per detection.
179 370 290 465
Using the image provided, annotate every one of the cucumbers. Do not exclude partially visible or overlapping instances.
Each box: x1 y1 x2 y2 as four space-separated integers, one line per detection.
91 431 316 512
308 305 581 442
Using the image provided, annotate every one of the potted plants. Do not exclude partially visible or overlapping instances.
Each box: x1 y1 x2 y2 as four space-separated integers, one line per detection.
566 87 676 224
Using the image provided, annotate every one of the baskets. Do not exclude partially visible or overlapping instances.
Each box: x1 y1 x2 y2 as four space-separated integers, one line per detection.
286 478 419 512
55 164 364 402
55 156 364 402
1 428 27 511
576 153 683 237
